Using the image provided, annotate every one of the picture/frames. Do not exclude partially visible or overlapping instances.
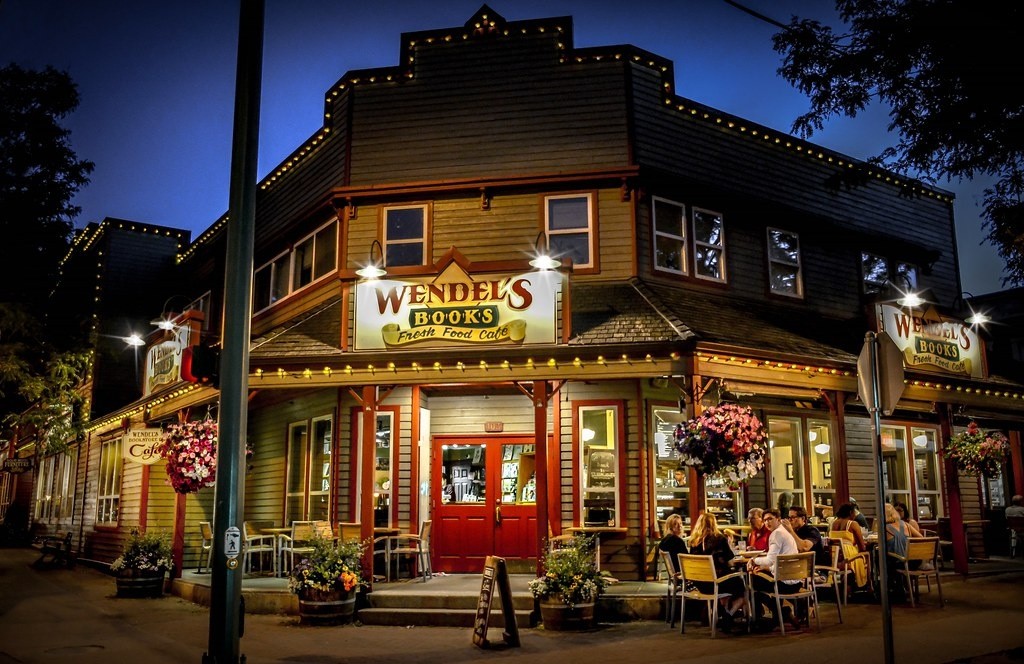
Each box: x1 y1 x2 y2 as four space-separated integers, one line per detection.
823 462 831 479
786 463 794 480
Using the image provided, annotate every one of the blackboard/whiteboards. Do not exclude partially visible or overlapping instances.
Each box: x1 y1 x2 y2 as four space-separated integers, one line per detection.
471 555 521 646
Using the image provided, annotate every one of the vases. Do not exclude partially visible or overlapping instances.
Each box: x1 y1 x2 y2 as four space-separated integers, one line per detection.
297 587 356 626
539 591 596 632
114 568 165 596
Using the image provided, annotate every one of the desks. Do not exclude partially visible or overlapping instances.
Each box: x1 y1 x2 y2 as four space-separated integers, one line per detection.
262 528 402 583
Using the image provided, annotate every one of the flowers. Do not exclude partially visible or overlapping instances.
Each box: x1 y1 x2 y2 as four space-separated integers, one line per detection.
673 401 770 490
529 533 618 609
110 525 175 572
155 419 255 493
936 422 1010 480
287 532 369 591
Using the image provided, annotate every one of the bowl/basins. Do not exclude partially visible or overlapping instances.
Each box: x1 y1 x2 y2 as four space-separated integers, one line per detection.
740 551 763 558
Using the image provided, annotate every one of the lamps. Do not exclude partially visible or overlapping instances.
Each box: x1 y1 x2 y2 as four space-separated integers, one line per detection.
529 231 562 269
877 274 926 306
913 432 927 446
809 428 830 454
954 292 992 323
355 240 387 278
151 296 193 329
122 319 151 346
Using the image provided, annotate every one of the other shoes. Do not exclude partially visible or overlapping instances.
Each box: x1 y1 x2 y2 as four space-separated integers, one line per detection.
792 615 800 629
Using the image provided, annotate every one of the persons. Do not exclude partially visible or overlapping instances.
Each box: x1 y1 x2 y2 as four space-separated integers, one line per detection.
747 507 775 557
746 509 802 629
658 514 697 590
1004 495 1024 541
849 496 870 531
781 506 828 621
442 473 456 501
672 468 690 513
829 504 874 599
893 502 922 601
871 502 923 602
686 513 746 634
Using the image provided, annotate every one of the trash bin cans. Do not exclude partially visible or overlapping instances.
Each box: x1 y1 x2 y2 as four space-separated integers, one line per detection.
935 517 970 562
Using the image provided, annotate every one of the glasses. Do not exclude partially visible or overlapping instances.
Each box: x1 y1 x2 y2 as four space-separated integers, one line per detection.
897 509 903 513
748 518 758 521
763 518 774 522
787 516 798 520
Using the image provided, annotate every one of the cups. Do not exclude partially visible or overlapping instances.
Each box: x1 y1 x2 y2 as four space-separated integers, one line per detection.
826 517 834 524
810 516 817 524
738 541 746 552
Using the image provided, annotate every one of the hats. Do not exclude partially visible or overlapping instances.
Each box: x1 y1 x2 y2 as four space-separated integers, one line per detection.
849 497 855 503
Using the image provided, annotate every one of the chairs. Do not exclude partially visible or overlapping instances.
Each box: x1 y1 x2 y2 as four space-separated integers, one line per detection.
658 519 943 639
199 520 433 583
